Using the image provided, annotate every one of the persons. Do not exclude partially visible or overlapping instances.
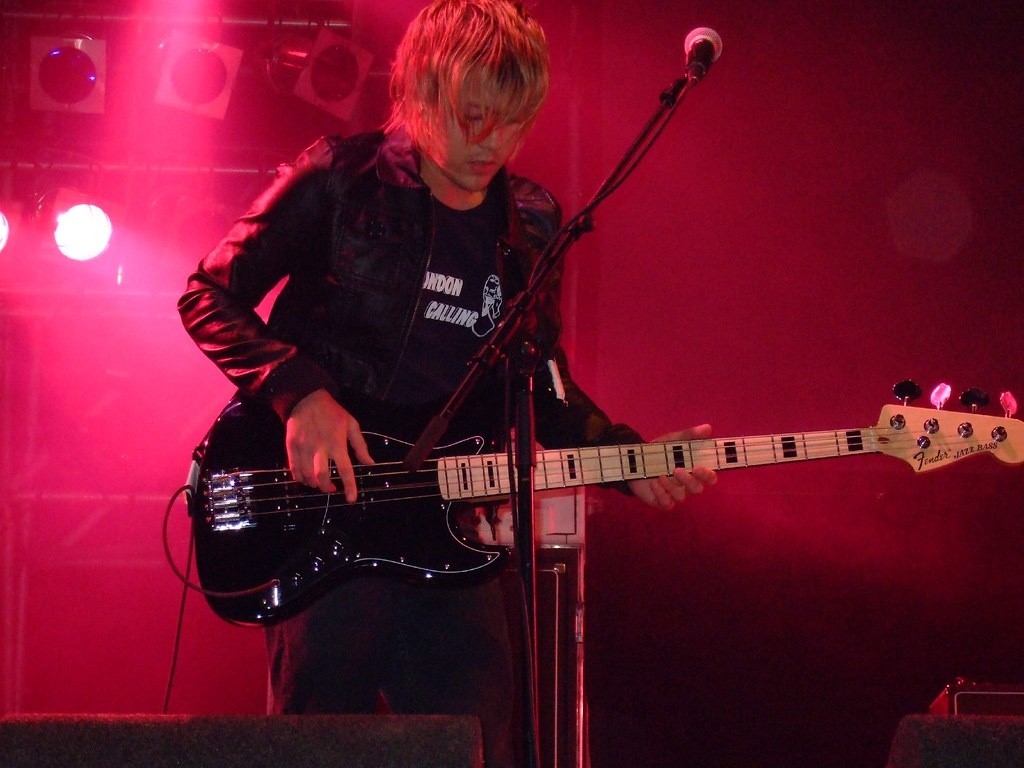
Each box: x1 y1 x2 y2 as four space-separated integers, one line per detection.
175 0 719 768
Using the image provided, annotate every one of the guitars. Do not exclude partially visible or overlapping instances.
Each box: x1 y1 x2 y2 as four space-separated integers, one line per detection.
194 377 1024 628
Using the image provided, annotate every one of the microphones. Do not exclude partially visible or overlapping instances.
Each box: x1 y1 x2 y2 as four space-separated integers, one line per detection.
683 25 724 81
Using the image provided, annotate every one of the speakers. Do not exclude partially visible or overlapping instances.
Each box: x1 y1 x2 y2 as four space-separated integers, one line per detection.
886 711 1024 768
0 714 487 768
